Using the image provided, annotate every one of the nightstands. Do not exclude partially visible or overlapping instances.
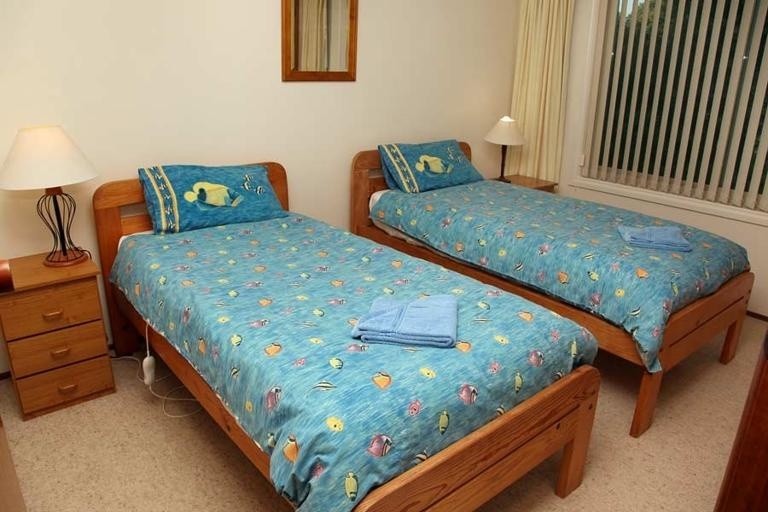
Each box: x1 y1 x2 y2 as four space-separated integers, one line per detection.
487 175 558 194
0 247 117 422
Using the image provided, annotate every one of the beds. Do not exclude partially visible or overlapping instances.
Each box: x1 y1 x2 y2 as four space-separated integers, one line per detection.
351 142 754 438
93 162 602 511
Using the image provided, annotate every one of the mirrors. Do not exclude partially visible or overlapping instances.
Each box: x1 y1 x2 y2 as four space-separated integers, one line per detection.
281 0 359 82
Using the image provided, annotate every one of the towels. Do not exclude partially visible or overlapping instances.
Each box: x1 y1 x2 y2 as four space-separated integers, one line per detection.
352 295 458 349
616 223 696 253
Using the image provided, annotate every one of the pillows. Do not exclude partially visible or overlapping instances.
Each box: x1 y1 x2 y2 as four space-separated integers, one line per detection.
377 139 484 194
138 165 288 234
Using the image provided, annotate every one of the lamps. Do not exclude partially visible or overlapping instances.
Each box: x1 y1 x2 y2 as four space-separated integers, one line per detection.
483 116 526 185
1 126 95 269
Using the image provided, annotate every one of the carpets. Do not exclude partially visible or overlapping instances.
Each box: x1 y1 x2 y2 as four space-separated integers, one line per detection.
0 309 768 510
